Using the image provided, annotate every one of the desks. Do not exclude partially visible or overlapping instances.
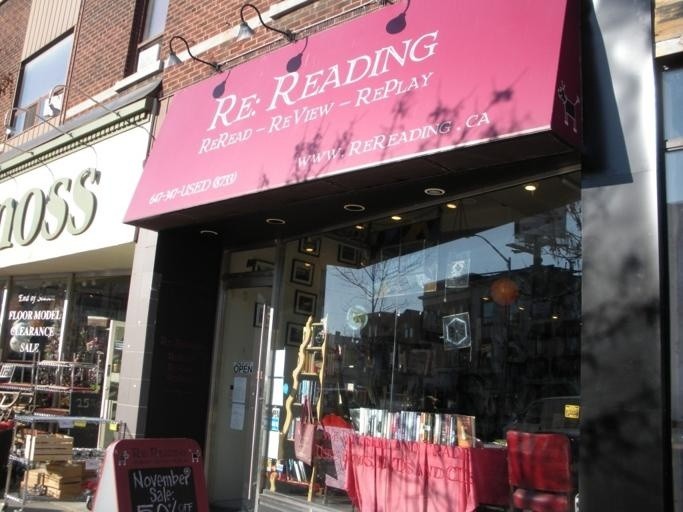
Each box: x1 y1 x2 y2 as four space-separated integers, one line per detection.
325 425 507 512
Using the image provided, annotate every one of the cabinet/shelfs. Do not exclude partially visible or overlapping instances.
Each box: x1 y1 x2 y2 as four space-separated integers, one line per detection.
270 315 376 504
0 294 131 512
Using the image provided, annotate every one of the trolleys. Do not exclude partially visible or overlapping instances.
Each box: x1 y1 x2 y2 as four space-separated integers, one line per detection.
0 414 127 511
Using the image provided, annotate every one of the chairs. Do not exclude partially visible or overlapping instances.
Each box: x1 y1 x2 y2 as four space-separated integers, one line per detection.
321 414 356 512
505 430 578 512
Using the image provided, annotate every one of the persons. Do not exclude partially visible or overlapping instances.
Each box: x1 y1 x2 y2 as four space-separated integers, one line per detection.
71 334 102 387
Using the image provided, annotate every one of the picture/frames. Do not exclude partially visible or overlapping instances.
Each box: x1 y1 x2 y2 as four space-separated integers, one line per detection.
298 234 322 258
337 243 357 265
293 289 318 318
253 259 275 272
253 302 280 330
285 321 306 348
290 258 315 287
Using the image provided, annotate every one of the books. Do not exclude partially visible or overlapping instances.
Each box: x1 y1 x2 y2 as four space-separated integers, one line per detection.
282 348 322 484
346 406 477 451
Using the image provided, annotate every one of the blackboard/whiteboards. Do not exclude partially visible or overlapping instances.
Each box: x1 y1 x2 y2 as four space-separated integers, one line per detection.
114 438 210 512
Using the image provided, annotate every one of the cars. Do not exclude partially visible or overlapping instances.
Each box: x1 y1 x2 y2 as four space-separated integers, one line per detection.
502 396 580 461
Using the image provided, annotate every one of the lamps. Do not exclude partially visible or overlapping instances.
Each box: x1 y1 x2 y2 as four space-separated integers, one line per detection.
41 84 120 117
0 140 34 160
236 4 295 45
2 106 72 128
166 36 220 73
